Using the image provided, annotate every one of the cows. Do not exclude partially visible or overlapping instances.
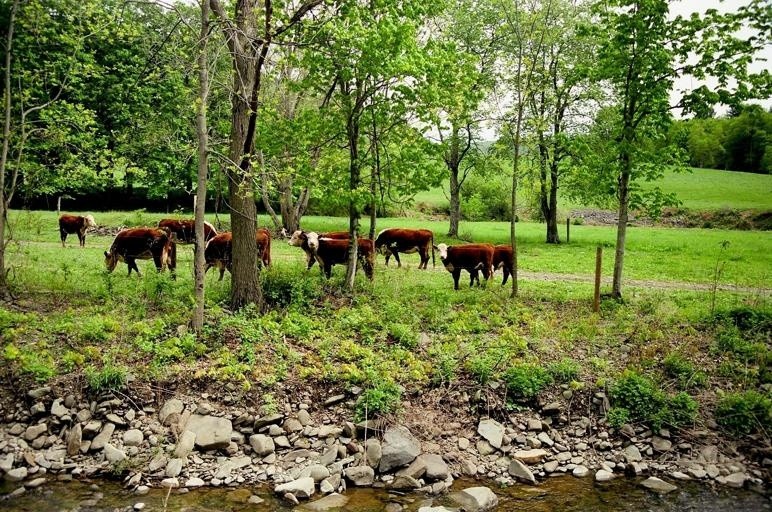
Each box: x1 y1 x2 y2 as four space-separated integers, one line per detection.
191 228 274 281
431 242 495 291
159 217 218 246
467 243 515 287
58 214 96 247
288 227 365 274
372 227 435 269
302 230 376 282
104 226 180 277
